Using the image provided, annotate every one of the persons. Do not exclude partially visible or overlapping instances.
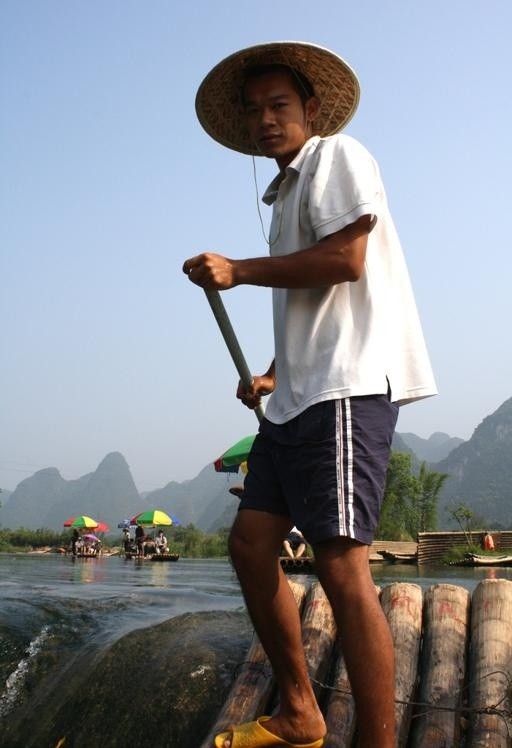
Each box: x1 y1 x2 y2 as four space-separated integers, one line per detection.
481 532 494 551
71 528 102 557
183 42 439 746
282 526 307 557
122 528 169 558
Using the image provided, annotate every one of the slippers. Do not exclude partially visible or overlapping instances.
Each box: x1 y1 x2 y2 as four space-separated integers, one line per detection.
214 715 324 748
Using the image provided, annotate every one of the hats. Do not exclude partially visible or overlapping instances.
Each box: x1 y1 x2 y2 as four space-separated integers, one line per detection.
195 42 361 156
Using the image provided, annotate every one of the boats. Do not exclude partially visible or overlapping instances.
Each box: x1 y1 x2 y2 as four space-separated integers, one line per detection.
77 553 98 557
132 553 179 560
366 530 512 567
126 551 138 559
201 578 511 747
277 556 316 575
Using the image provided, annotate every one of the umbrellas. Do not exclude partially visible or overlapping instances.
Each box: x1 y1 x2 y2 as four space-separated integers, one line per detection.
80 521 109 534
64 515 99 528
118 518 139 529
213 435 257 473
130 505 181 539
